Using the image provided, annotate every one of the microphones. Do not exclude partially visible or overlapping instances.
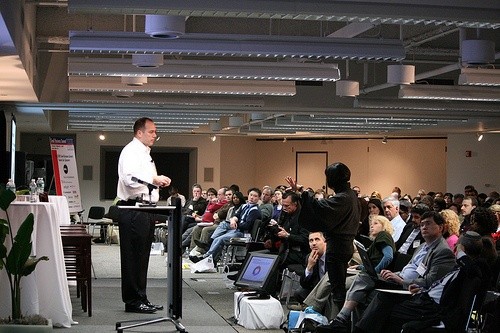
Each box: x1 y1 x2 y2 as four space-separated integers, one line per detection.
131 177 157 189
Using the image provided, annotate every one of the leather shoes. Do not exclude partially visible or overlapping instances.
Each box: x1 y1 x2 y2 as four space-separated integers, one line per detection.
125 303 163 313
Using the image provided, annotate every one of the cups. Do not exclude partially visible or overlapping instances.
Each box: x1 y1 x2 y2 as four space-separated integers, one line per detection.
218 267 223 273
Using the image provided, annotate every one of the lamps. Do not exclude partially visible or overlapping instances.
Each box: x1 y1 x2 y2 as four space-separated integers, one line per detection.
461 29 496 63
386 63 416 85
144 14 185 38
335 80 360 98
132 55 163 67
209 134 216 141
229 116 243 128
122 76 148 86
210 121 221 132
382 136 388 143
476 131 483 142
251 113 266 121
283 137 288 142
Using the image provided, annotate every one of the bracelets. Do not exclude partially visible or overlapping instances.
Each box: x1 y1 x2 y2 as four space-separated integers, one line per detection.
298 185 304 191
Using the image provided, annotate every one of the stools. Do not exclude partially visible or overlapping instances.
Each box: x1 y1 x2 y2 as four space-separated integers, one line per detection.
59 223 93 317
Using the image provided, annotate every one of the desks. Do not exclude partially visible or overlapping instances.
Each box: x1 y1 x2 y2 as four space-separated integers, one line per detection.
15 194 71 225
0 201 78 327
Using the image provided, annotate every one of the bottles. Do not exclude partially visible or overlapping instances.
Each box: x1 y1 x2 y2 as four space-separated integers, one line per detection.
6 179 16 194
29 178 38 195
36 177 45 194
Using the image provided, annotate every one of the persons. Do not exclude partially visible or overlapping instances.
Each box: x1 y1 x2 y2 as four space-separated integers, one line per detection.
182 184 261 267
167 187 185 208
306 185 381 200
33 168 45 183
285 161 361 321
92 196 121 244
258 185 287 231
227 191 396 333
381 187 500 333
464 185 473 197
117 116 171 312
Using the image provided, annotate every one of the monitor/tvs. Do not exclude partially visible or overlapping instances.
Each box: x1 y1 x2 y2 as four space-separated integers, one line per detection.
235 252 280 291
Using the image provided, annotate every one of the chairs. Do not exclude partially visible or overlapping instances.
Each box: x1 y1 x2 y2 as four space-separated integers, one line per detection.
87 200 121 246
216 216 500 333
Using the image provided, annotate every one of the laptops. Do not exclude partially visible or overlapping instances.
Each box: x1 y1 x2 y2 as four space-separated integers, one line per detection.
353 242 399 286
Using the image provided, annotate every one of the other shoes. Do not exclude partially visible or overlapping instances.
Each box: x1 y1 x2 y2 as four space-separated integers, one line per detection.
189 255 204 263
316 317 345 327
227 273 238 280
194 239 209 251
287 303 307 311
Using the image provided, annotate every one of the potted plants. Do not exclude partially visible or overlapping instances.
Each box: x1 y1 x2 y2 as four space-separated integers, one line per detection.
0 189 53 333
